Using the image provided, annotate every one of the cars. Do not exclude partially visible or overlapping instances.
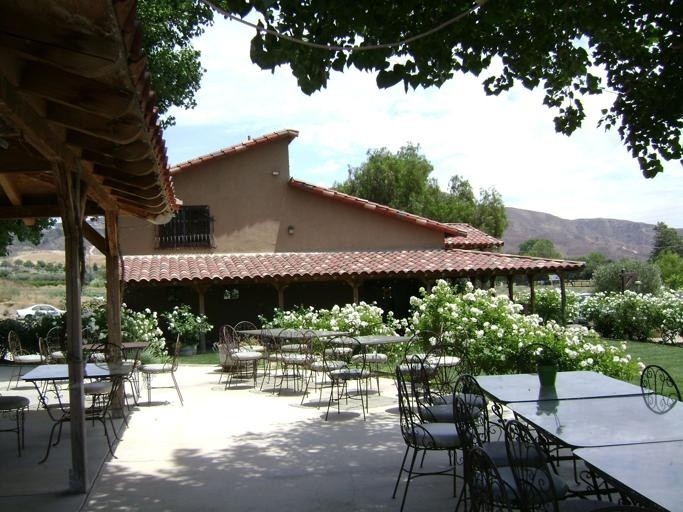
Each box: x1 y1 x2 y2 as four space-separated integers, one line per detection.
14 303 66 324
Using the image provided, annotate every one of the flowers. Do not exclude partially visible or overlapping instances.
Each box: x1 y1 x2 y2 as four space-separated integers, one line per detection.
159 303 214 344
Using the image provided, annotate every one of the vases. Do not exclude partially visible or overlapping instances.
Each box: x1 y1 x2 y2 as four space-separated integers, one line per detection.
180 344 197 355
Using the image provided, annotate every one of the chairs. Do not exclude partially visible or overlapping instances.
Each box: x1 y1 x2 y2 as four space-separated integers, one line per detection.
218 322 398 423
0 326 184 457
640 364 682 403
392 332 624 510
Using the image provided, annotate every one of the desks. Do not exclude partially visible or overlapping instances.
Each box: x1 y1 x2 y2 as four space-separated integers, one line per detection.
237 326 348 340
325 335 417 344
472 370 683 511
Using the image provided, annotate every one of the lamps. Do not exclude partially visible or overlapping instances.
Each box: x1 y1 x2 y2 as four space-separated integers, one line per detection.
288 226 294 235
